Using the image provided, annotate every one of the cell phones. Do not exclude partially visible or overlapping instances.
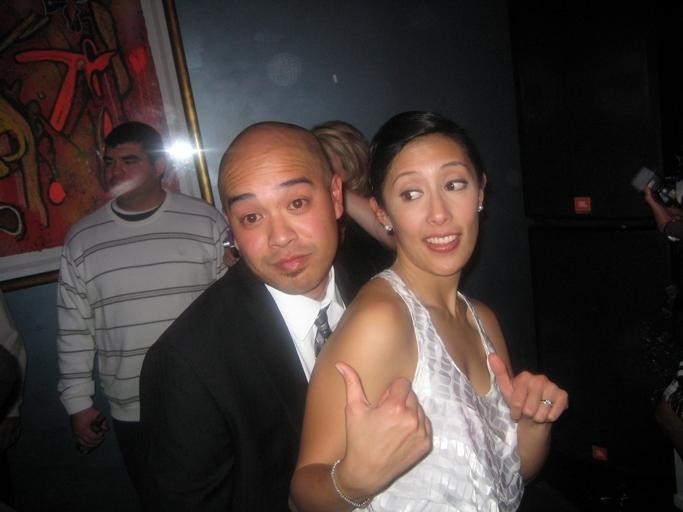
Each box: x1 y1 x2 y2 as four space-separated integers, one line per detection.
629 167 677 206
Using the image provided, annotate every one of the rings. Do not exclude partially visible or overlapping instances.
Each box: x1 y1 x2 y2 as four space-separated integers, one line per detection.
540 399 553 408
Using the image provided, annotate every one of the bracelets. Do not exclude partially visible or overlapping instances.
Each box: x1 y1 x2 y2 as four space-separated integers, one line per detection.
329 458 373 508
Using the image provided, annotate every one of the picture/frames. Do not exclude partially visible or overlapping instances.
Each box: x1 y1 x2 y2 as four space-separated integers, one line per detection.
0 0 216 296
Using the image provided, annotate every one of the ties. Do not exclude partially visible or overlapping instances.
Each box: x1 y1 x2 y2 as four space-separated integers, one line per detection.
314 304 333 356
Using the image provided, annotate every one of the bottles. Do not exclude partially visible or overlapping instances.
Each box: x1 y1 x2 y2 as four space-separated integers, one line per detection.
76 407 109 456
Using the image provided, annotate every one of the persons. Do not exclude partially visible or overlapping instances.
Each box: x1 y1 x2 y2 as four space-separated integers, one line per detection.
634 151 682 241
132 118 394 511
52 122 241 512
282 106 572 511
0 286 29 475
220 120 398 313
